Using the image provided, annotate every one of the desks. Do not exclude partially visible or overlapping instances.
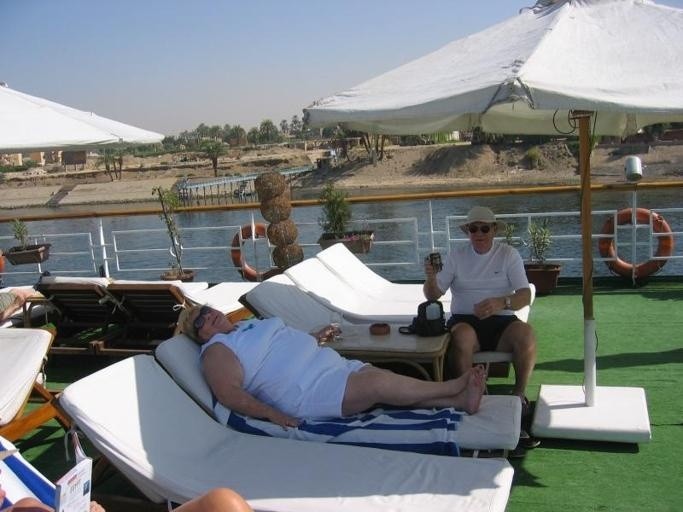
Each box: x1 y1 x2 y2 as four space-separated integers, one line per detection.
308 324 451 382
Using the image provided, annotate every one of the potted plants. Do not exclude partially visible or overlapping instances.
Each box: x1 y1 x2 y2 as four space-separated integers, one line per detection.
526 217 561 294
152 187 199 281
2 219 51 265
317 175 375 254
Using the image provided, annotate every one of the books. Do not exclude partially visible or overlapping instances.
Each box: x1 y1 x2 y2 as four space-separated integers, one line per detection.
53 430 95 512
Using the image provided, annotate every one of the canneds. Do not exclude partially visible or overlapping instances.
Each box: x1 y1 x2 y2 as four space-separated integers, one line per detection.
430 253 442 272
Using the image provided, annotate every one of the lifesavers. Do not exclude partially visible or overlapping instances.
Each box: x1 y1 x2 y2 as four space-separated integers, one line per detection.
599 208 672 277
231 223 283 281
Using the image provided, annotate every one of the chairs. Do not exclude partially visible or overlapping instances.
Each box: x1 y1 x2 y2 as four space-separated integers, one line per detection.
96 281 262 354
316 242 536 306
23 271 209 354
0 436 56 512
0 285 61 328
238 273 525 394
59 353 515 512
283 257 530 324
155 333 522 458
0 323 72 443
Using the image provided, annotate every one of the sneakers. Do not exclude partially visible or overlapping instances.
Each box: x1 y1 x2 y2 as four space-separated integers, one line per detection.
522 397 528 416
511 430 541 457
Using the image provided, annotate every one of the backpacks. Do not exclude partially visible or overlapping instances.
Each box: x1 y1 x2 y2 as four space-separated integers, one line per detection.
398 300 446 337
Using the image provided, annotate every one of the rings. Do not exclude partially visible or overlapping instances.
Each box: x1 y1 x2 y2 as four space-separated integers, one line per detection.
484 310 488 313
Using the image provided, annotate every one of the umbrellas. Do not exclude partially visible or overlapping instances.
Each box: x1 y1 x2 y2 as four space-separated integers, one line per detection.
0 81 166 154
304 0 682 405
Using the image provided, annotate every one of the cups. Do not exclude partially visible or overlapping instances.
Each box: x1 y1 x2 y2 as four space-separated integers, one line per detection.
331 311 345 343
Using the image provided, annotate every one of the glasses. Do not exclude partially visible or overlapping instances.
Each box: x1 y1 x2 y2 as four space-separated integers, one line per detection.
467 224 492 234
193 305 209 337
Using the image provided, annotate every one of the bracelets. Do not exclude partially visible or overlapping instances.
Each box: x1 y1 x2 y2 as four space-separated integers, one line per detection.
503 295 512 309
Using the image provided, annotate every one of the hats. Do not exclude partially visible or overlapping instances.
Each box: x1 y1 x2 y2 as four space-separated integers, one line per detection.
457 206 504 235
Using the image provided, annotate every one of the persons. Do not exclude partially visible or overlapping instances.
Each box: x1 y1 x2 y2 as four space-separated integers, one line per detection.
0 288 35 325
177 304 486 432
0 447 255 512
423 206 538 418
